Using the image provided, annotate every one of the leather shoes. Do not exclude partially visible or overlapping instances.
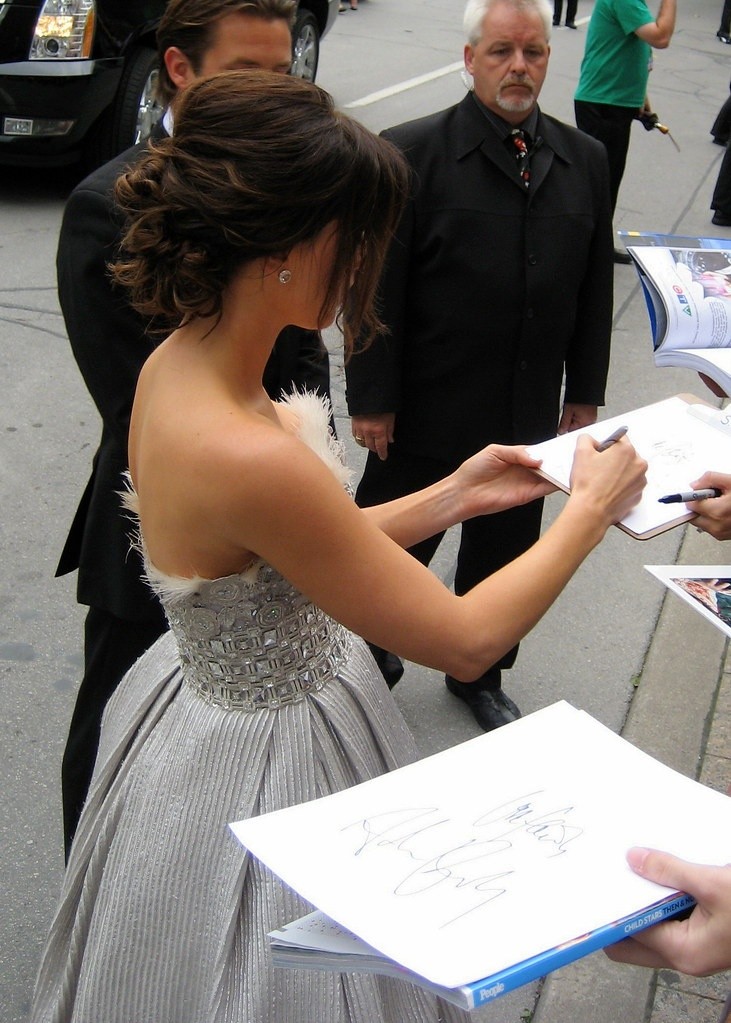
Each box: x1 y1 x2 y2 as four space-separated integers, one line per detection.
444 672 521 733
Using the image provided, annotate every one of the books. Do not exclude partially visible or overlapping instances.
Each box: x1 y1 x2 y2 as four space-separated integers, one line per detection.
617 230 731 400
226 698 731 1010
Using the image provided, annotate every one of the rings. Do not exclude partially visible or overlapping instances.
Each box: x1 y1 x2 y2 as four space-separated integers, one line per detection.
355 436 364 441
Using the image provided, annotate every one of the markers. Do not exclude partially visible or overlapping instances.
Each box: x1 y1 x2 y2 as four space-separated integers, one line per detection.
658 488 720 503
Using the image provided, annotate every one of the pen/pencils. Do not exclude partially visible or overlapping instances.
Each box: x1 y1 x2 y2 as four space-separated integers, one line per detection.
597 424 628 452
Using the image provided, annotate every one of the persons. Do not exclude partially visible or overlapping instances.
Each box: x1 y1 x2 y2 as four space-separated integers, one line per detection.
572 0 678 263
604 845 730 980
27 69 648 1019
56 0 332 865
716 0 731 44
552 0 577 29
685 470 731 541
342 0 615 731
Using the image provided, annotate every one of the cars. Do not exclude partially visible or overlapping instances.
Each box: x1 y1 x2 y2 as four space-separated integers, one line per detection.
0 0 339 165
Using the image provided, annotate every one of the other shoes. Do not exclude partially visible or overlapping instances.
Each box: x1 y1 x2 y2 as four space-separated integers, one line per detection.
717 31 731 43
552 19 560 26
612 247 633 262
564 21 577 29
711 208 731 228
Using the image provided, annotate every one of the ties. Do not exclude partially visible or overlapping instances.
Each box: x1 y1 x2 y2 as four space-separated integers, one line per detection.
511 127 532 188
